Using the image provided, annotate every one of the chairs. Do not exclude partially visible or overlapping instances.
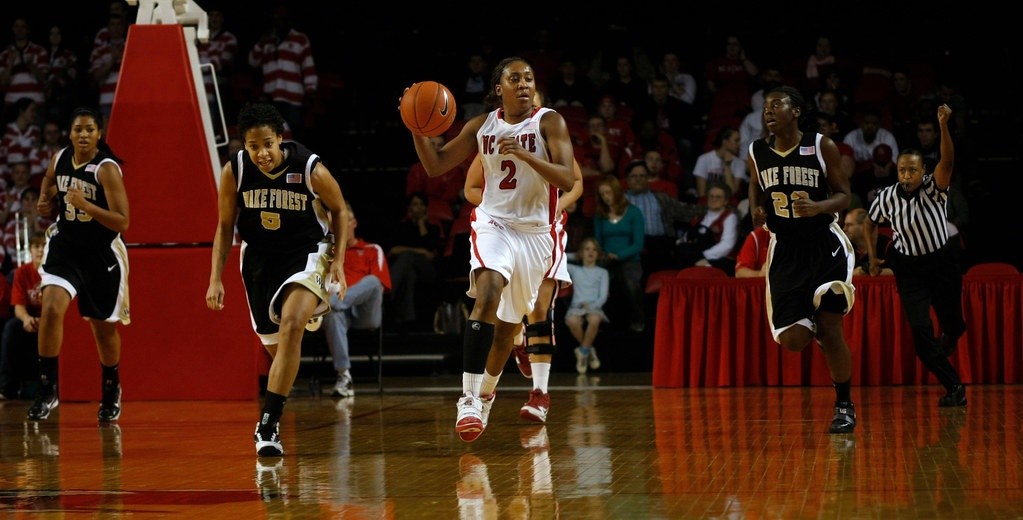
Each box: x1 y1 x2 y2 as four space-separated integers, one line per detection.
312 223 685 396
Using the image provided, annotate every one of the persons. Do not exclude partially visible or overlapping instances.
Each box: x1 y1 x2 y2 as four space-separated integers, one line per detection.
464 89 584 423
746 82 859 435
205 101 350 474
26 110 133 425
388 17 981 374
322 206 392 397
863 102 982 408
0 0 318 400
398 57 575 443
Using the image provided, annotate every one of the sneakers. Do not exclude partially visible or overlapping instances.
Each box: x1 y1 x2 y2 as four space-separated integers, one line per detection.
588 347 600 370
331 374 354 396
830 433 856 457
458 454 493 504
27 391 59 421
255 409 284 456
512 322 533 379
456 391 484 433
29 434 59 460
516 459 533 496
574 348 588 374
829 402 857 434
940 383 968 407
256 454 283 503
97 424 123 459
331 395 354 416
520 423 551 453
97 384 122 422
936 320 966 356
520 388 550 423
941 408 967 428
459 390 496 442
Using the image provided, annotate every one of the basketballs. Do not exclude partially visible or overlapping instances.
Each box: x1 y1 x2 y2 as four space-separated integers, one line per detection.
399 80 458 138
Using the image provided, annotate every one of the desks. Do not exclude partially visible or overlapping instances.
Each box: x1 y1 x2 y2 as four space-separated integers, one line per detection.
651 274 1023 389
